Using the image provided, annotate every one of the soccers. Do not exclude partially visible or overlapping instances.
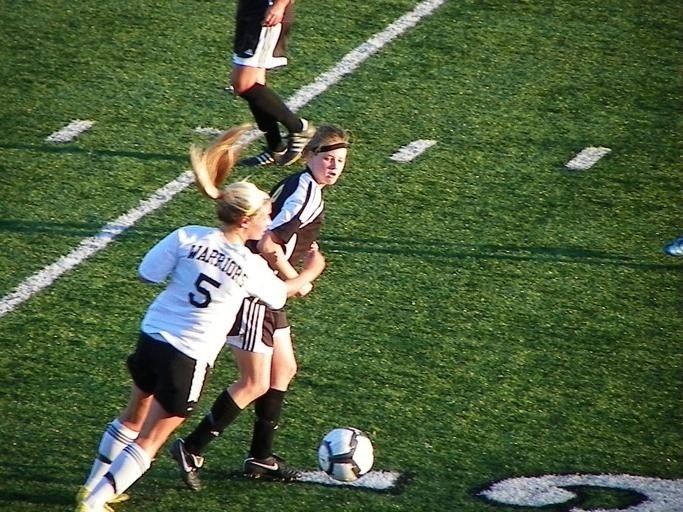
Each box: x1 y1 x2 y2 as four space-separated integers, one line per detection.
318 424 374 480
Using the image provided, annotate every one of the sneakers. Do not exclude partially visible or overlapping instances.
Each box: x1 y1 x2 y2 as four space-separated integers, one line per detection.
74 500 114 512
243 455 302 484
237 147 288 168
74 484 130 504
278 117 316 167
171 436 202 492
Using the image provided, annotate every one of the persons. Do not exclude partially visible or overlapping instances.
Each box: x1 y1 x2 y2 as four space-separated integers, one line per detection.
230 0 316 167
170 124 348 492
75 182 325 512
